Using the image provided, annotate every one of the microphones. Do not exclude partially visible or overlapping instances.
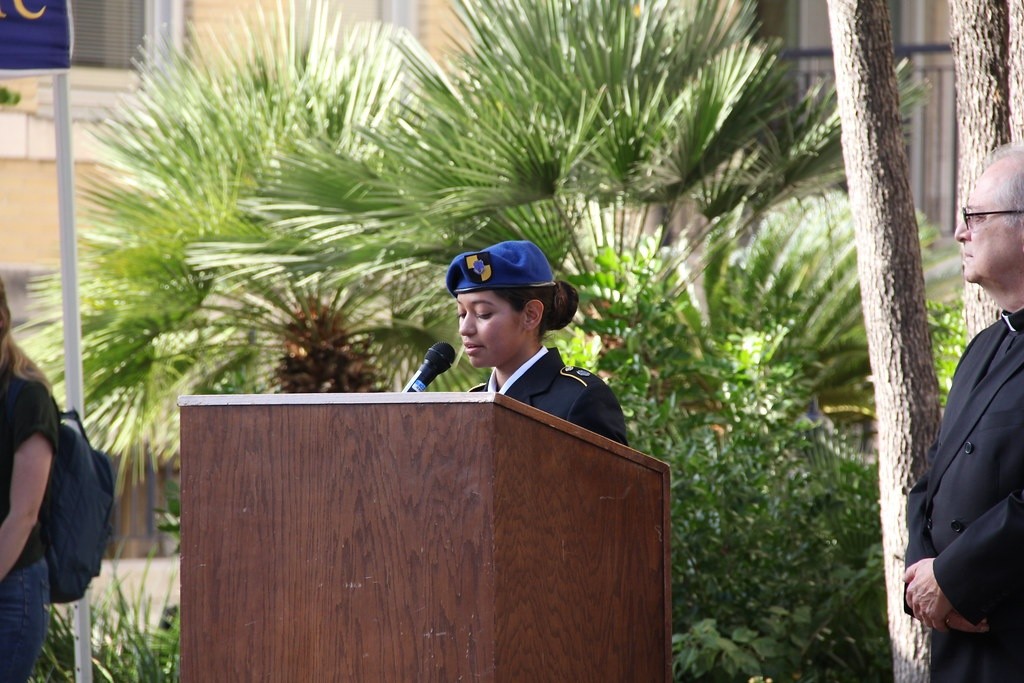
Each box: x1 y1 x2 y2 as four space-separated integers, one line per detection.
403 343 455 393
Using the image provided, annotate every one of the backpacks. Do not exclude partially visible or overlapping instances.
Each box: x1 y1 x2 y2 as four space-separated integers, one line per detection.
41 407 116 605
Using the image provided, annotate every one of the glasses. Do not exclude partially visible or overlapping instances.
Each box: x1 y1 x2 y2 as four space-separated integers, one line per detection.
962 207 1024 231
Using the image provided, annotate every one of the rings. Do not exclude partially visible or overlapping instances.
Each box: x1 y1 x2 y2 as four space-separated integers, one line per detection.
944 617 949 625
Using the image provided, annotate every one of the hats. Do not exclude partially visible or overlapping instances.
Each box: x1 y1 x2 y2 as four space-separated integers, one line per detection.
444 240 557 299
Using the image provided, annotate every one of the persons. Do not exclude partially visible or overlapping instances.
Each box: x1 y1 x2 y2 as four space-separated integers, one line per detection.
446 241 630 447
899 140 1024 683
0 279 60 683
